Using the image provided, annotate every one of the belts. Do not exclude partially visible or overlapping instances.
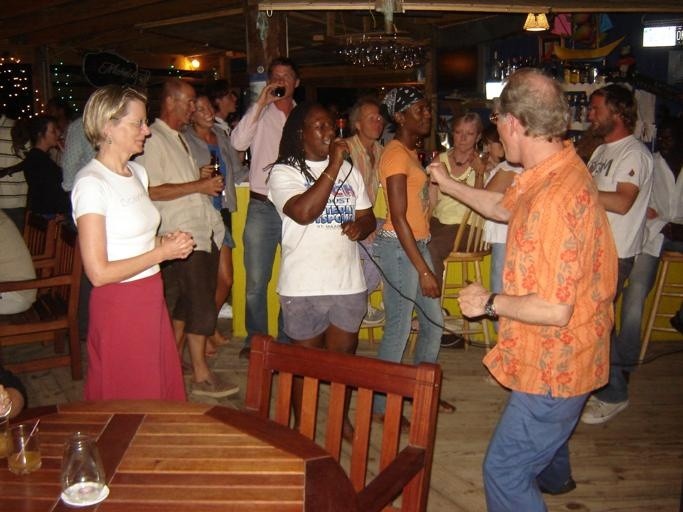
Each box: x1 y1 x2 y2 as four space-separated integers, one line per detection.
249 190 269 203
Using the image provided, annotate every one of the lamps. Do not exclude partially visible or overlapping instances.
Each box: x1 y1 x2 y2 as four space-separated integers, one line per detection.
334 1 425 75
190 56 200 69
522 12 550 31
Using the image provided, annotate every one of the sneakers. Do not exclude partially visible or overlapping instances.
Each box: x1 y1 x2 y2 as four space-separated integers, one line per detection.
208 334 230 344
540 480 575 494
203 346 219 358
373 413 410 433
191 373 239 398
437 397 455 414
239 344 250 359
581 396 630 424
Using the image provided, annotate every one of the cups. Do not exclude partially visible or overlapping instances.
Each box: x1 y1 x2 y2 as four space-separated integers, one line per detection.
3 423 42 476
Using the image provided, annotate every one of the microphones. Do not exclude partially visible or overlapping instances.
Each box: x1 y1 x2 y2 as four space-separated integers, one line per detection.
343 152 353 165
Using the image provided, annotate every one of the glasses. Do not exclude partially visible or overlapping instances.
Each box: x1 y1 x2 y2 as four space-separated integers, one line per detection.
110 116 147 127
489 113 503 124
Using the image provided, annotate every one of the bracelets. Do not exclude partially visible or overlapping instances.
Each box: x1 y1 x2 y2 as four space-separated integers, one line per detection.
159 235 164 245
320 171 335 183
478 151 487 159
418 270 435 280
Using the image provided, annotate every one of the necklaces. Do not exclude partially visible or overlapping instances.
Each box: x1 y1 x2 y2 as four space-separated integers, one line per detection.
451 151 472 168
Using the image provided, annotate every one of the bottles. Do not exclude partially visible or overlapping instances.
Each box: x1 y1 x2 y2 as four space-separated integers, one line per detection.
491 50 500 80
571 65 580 83
590 63 599 83
580 63 590 83
517 55 524 67
211 156 222 195
578 93 588 123
500 60 505 80
525 56 530 66
571 95 577 121
505 64 511 76
569 133 576 145
567 94 571 106
563 63 570 83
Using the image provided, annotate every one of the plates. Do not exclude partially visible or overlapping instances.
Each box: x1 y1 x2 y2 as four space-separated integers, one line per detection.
61 482 110 508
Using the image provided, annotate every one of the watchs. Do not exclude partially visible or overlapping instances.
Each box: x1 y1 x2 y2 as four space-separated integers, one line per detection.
484 292 498 320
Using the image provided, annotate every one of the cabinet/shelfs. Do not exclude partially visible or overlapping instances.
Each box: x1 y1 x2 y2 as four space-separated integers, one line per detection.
555 44 615 138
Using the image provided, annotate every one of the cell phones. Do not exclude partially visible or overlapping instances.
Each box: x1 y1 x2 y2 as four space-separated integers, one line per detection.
641 21 683 46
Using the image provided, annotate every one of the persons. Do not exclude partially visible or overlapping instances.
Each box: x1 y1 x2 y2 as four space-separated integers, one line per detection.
617 153 675 385
580 83 655 423
61 103 99 211
70 83 196 401
0 97 75 180
231 58 300 359
475 122 525 337
0 211 39 315
12 114 71 216
204 78 246 162
371 85 457 434
429 103 495 296
185 91 250 359
264 101 377 446
134 78 240 397
456 72 616 512
342 102 386 325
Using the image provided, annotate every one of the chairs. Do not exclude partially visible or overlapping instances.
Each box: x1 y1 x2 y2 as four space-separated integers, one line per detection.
0 217 77 396
246 340 444 428
447 201 490 348
642 251 683 366
